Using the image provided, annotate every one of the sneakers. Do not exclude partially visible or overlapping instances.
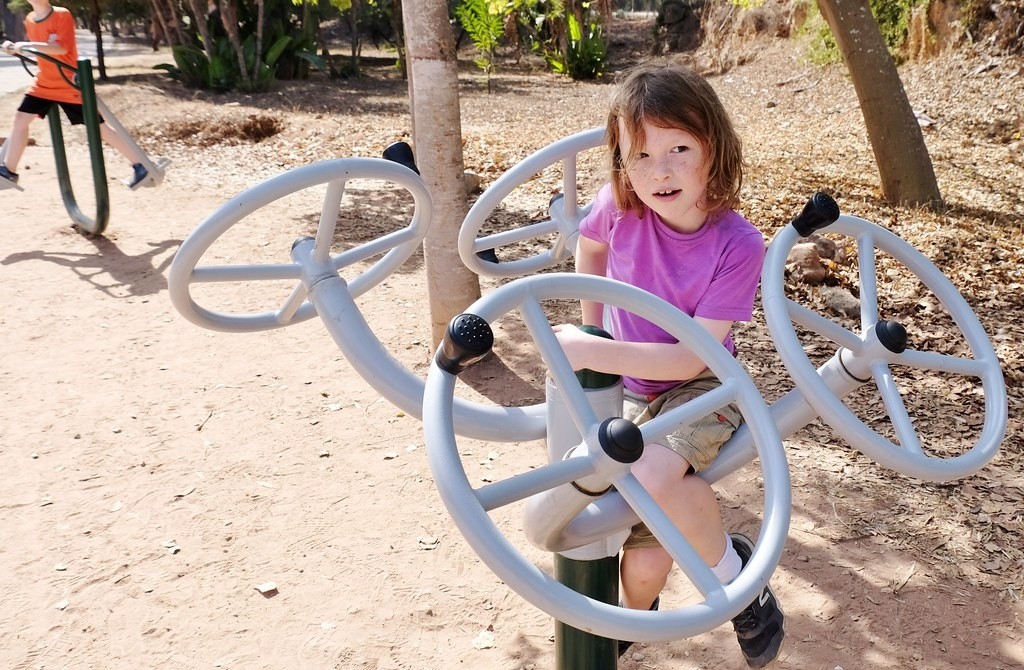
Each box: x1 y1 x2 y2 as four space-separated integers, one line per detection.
618 595 660 659
727 533 785 670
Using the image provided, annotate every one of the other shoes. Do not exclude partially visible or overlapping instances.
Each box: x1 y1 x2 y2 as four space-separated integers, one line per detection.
0 166 19 183
128 160 159 189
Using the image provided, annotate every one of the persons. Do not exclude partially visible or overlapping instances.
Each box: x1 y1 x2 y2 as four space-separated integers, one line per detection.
0 0 153 189
542 62 784 670
